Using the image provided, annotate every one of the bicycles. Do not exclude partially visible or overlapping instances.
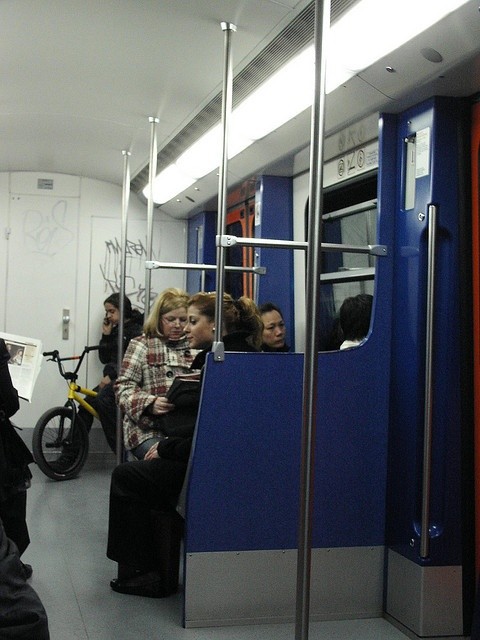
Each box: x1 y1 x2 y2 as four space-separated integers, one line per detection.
32 343 116 481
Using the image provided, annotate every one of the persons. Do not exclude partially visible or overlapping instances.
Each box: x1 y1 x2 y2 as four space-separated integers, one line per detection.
337 293 374 350
46 292 142 471
106 291 264 599
0 337 34 558
252 301 292 353
112 288 192 460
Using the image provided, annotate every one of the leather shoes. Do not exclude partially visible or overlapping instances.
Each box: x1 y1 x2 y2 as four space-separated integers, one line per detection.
110 578 163 598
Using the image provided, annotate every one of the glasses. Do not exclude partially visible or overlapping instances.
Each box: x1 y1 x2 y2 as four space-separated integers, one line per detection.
164 316 189 329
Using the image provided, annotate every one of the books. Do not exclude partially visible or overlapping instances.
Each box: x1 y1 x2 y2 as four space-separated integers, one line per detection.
163 372 202 409
